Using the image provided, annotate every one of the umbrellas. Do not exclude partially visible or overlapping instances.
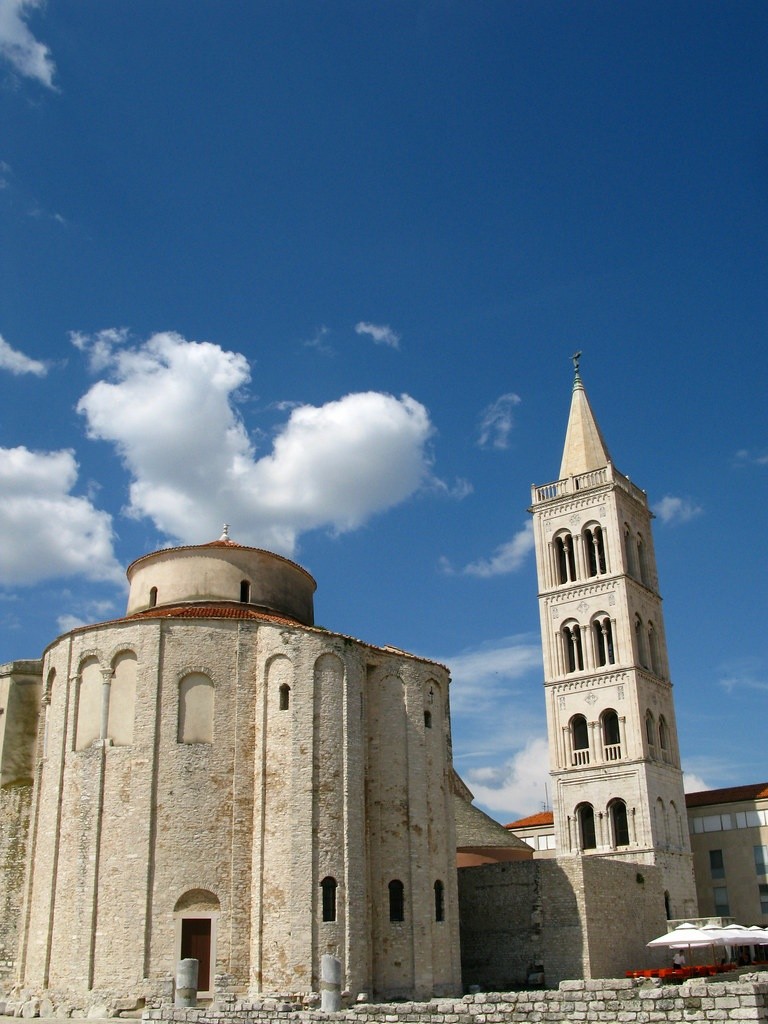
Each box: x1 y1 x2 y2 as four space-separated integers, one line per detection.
646 922 768 971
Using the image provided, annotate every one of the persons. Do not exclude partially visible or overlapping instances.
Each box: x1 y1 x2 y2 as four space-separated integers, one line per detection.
672 950 688 969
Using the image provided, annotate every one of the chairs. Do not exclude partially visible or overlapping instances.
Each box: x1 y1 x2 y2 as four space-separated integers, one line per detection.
626 964 737 985
